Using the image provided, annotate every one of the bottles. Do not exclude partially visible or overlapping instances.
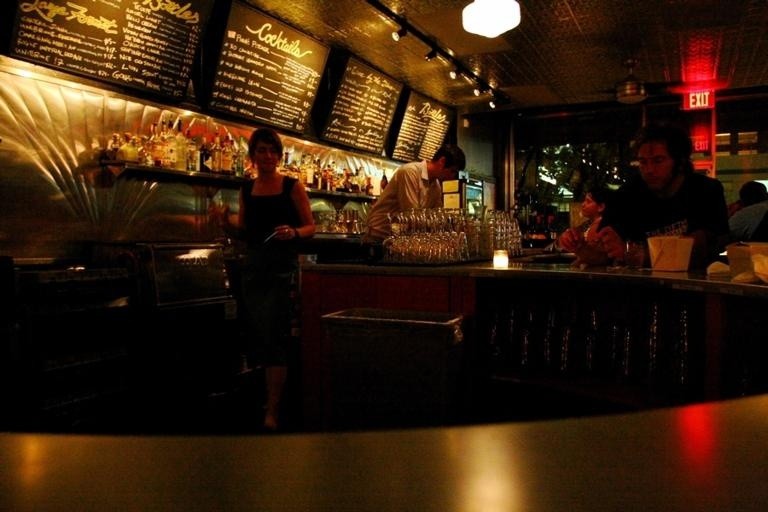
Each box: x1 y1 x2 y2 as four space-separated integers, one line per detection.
111 118 389 196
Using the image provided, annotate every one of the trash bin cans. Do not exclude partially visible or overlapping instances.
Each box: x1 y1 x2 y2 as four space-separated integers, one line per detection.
320 308 464 433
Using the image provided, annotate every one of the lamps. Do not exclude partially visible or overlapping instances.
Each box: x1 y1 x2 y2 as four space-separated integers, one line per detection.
378 9 513 110
614 59 651 103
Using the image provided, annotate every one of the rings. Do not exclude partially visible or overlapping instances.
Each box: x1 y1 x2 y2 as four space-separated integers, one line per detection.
285 229 289 232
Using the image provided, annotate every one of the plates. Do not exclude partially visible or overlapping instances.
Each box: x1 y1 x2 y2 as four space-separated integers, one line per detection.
13 257 55 265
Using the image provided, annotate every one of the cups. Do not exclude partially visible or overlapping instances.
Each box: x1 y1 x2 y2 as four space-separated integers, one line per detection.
468 210 523 259
391 207 469 262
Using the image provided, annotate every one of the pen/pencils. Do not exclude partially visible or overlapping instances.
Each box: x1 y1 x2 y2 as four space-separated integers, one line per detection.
264 231 279 243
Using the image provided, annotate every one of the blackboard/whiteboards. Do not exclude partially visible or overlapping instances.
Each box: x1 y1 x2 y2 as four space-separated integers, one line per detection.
1 0 215 105
390 88 456 162
205 0 331 136
320 57 405 156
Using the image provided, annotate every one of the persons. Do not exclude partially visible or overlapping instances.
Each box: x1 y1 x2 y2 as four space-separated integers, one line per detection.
577 185 613 231
557 120 731 268
206 128 316 431
739 180 767 208
361 144 466 264
